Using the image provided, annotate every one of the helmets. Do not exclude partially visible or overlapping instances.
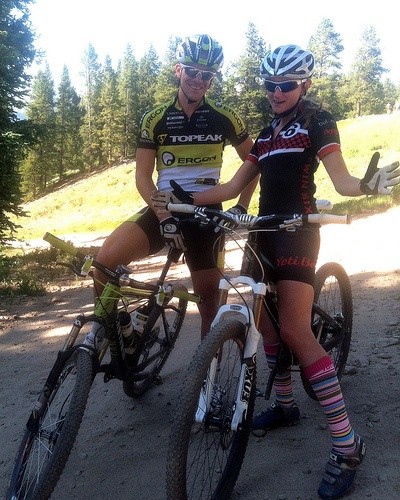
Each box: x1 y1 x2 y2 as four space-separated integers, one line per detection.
176 34 225 70
260 43 315 78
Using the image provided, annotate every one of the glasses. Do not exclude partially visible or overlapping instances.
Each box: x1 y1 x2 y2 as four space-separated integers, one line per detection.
264 78 308 93
179 63 214 82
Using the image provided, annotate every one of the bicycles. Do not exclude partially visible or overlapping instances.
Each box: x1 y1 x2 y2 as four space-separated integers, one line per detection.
6 232 204 500
165 199 353 500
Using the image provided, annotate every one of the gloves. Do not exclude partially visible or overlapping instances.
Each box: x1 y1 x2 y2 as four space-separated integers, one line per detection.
159 216 187 251
150 179 195 214
358 151 400 195
212 204 247 233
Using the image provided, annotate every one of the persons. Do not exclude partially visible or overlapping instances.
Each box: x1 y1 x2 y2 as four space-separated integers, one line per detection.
80 35 260 422
151 45 400 500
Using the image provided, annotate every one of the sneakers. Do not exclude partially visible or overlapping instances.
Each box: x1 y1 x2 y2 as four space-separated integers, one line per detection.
79 331 106 356
317 433 367 500
195 378 222 423
252 402 301 430
